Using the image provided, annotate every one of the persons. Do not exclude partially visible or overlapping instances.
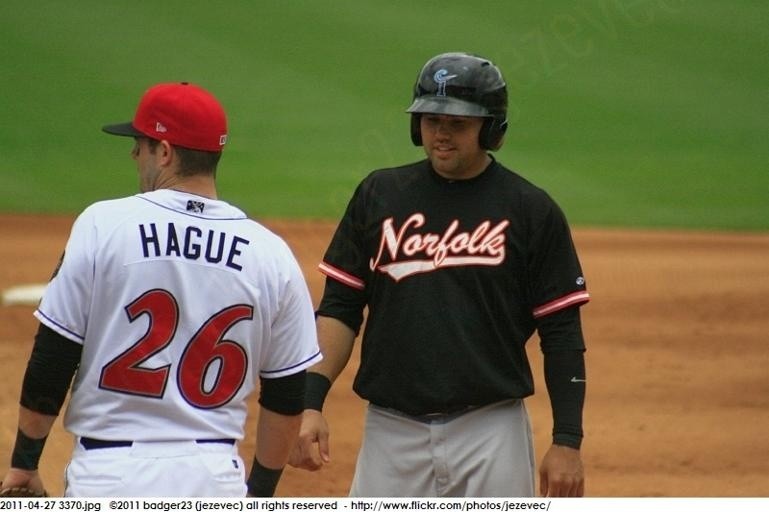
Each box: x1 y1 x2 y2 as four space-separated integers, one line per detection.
287 52 590 497
0 82 322 497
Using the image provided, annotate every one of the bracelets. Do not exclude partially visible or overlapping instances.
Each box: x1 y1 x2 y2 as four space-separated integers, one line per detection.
303 372 331 412
11 428 49 471
247 454 284 497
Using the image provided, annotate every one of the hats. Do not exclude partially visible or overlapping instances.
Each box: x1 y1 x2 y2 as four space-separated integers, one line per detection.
101 82 228 153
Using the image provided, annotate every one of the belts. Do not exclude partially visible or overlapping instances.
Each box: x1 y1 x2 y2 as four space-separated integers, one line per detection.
79 436 236 450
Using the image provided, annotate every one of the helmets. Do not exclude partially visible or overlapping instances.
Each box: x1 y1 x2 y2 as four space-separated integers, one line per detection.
405 51 508 151
